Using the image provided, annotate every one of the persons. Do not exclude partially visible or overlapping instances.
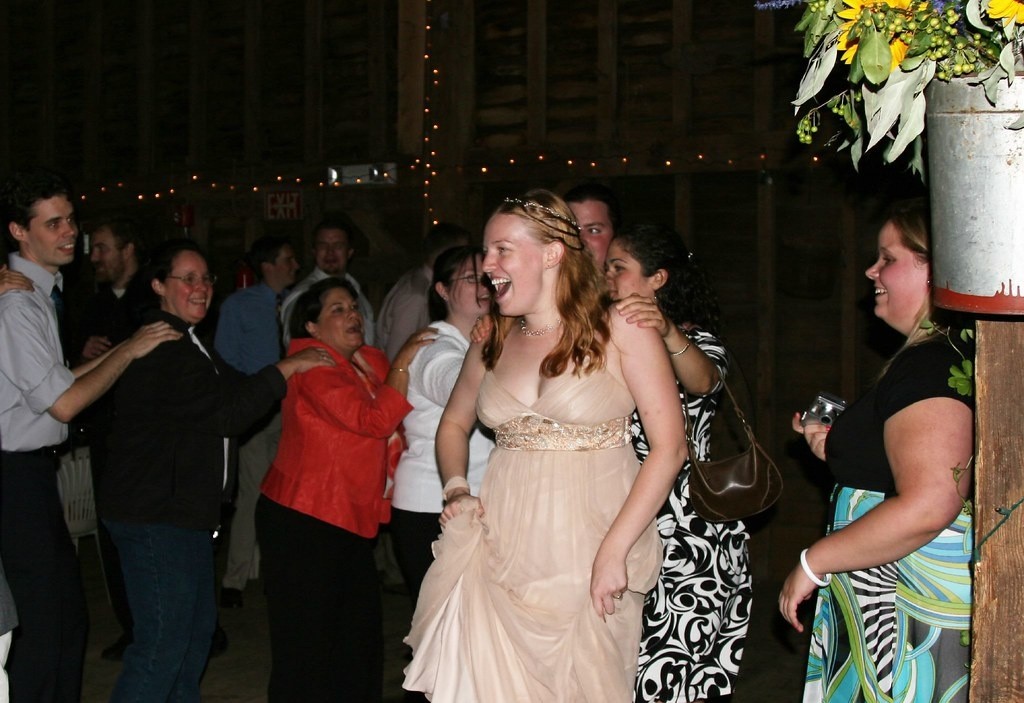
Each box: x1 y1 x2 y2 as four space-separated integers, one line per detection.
0 183 753 703
402 188 689 703
779 196 975 703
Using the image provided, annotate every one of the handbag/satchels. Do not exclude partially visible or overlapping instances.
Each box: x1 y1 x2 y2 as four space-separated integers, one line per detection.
680 366 787 525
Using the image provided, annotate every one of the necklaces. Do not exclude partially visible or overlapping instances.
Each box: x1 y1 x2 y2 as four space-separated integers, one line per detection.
520 318 561 337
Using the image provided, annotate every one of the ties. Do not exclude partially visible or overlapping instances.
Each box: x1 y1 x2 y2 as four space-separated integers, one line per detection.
274 293 287 359
50 284 76 370
189 326 229 490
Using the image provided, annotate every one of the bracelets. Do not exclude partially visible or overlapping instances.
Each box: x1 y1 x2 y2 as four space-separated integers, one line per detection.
443 492 470 507
669 339 692 358
800 549 832 586
443 475 470 500
389 368 408 374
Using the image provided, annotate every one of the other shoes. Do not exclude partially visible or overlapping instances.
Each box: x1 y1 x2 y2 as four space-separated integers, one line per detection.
209 622 230 659
218 586 245 609
382 581 410 598
101 629 137 662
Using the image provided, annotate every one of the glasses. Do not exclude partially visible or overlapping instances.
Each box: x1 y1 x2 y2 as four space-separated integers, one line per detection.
165 273 217 287
445 274 482 285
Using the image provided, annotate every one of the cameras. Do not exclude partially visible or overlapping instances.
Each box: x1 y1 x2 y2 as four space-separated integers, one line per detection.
801 391 848 427
